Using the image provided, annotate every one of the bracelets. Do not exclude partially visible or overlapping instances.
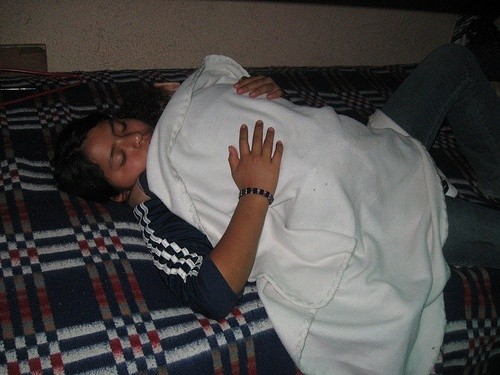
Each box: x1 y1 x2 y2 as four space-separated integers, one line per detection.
238 188 274 204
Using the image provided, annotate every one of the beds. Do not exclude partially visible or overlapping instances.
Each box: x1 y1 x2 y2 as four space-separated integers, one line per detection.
1 62 500 375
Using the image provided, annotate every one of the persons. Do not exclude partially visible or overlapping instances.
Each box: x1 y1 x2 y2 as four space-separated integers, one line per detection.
126 78 459 208
49 43 500 322
